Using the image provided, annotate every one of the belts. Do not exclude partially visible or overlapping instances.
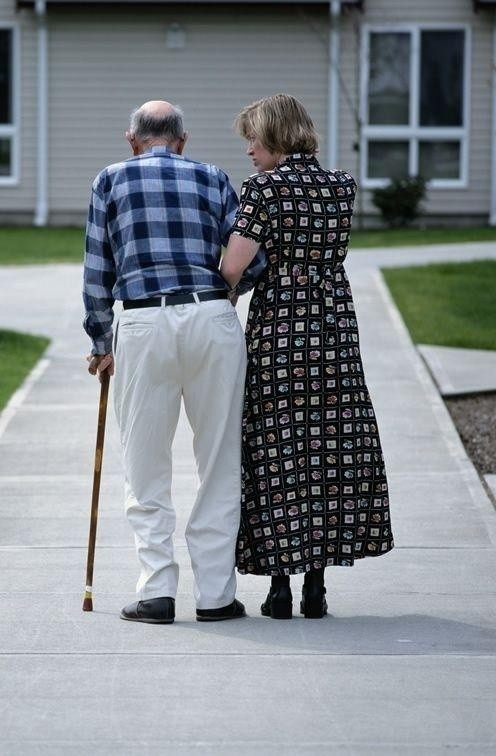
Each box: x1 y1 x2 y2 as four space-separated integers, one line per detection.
122 289 228 310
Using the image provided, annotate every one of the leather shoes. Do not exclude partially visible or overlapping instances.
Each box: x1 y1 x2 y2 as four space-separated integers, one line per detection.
195 598 245 620
119 596 175 623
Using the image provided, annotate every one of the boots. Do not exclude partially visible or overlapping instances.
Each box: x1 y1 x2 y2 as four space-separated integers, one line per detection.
300 567 327 618
260 575 292 619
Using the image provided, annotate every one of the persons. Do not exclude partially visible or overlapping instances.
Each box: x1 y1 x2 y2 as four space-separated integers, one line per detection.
82 99 272 628
219 91 398 621
